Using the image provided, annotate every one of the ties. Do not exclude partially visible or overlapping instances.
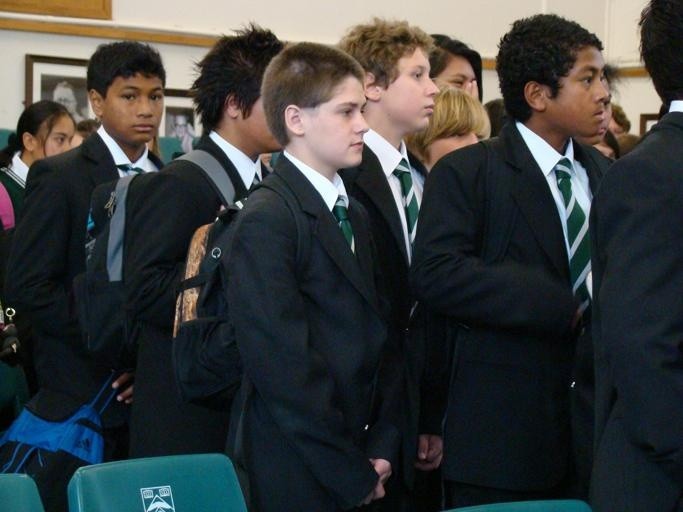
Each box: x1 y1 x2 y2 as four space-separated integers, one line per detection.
334 193 354 250
552 159 592 341
392 158 419 261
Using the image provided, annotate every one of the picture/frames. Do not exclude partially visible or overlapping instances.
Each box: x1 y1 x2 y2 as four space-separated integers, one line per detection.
24 53 202 139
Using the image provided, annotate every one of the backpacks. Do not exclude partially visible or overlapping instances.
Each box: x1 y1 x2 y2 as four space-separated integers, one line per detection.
168 174 313 401
75 171 147 350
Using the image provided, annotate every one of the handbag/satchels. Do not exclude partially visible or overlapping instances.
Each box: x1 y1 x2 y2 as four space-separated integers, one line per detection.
1 377 113 512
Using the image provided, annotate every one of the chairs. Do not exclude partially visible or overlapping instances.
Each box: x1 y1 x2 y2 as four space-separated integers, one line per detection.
2 452 248 507
